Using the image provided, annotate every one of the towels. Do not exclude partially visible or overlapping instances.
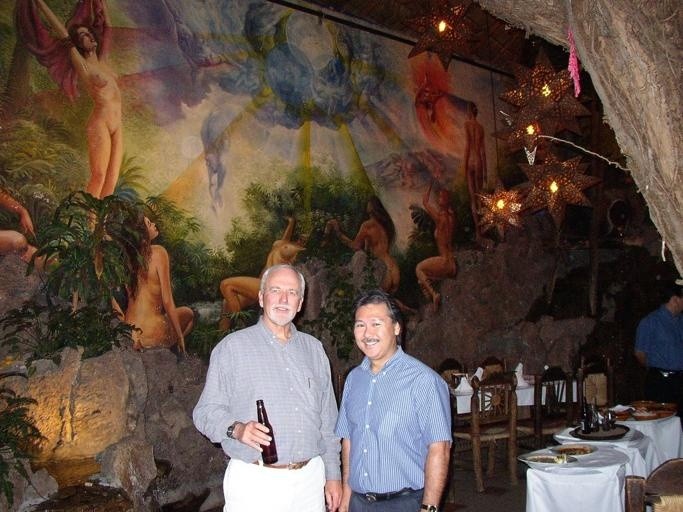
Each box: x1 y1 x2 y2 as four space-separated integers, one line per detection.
515 364 528 387
607 404 636 412
456 377 473 392
554 427 581 441
471 366 484 382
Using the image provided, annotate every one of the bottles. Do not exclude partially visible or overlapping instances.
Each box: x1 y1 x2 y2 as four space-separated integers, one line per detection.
581 396 590 433
590 396 599 433
255 399 278 465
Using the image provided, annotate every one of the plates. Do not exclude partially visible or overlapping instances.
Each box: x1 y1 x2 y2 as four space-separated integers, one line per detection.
611 399 677 420
574 424 630 440
516 451 578 465
548 443 598 456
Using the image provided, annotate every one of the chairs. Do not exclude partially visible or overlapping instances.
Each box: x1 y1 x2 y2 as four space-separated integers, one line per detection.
625 457 683 512
451 371 519 493
576 358 614 424
518 364 574 454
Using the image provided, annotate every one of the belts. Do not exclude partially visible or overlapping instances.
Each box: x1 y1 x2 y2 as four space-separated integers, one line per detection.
352 485 415 504
250 456 314 471
647 369 682 378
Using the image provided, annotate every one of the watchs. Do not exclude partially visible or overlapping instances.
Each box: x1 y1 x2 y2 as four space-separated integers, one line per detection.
227 422 237 439
420 504 439 512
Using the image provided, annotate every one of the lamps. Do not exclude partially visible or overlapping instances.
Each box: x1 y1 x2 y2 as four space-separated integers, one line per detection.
512 152 601 229
497 47 591 136
401 1 477 71
474 184 527 242
499 115 559 164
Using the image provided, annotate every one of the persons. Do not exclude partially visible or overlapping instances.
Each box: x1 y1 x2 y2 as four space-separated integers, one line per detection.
219 217 308 330
193 264 343 510
0 182 54 271
415 176 457 307
116 215 193 360
33 0 124 230
336 287 453 512
633 278 682 418
463 103 485 243
334 196 414 316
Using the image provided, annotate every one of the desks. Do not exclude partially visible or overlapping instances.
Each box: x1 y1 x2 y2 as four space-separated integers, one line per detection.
554 423 667 480
524 445 646 512
596 405 683 465
445 378 580 479
